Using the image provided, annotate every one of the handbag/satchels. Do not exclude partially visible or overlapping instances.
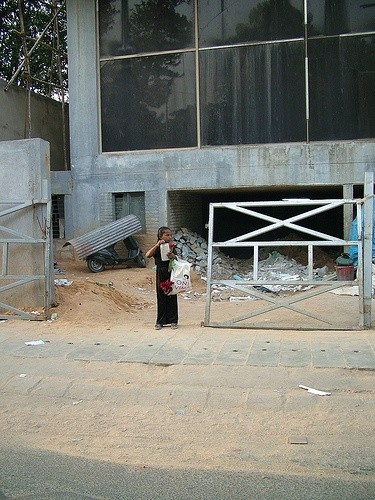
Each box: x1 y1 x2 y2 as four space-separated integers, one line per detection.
167 255 192 296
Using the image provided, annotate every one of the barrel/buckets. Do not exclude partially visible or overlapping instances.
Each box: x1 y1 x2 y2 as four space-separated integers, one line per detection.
336 265 354 281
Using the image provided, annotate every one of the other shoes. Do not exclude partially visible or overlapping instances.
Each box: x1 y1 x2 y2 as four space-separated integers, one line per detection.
171 323 179 329
154 323 163 330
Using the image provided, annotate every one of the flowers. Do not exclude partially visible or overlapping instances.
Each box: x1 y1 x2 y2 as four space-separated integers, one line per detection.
160 279 174 295
168 244 176 273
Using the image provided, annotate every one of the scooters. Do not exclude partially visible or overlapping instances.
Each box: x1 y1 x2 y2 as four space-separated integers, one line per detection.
82 235 146 273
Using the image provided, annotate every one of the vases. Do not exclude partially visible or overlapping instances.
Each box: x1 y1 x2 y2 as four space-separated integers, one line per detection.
334 265 354 281
335 253 354 266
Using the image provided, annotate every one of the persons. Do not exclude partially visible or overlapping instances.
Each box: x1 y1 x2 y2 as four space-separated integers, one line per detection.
145 226 179 330
